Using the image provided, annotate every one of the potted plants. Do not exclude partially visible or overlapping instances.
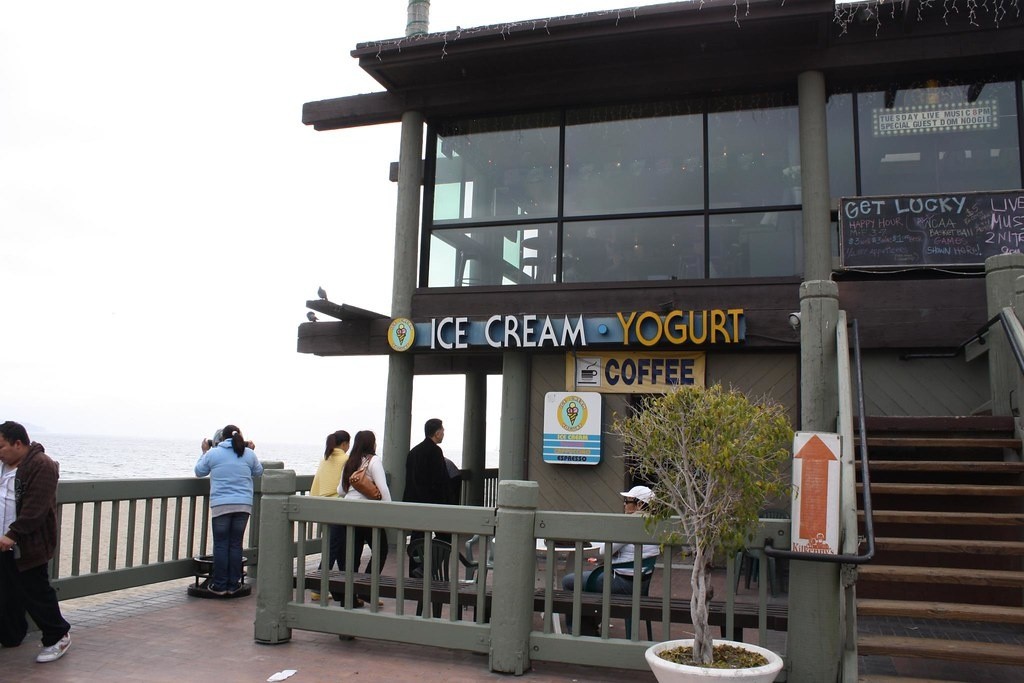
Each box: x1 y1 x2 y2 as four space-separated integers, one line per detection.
601 375 799 683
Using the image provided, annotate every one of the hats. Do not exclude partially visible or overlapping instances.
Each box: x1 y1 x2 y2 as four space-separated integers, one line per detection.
620 486 657 504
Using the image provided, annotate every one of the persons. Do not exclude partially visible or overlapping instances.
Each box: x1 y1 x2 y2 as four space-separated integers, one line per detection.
561 486 661 637
310 431 350 601
337 430 391 609
194 424 263 595
0 421 72 662
402 419 462 579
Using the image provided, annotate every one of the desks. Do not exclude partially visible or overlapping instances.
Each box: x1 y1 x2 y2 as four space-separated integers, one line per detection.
696 224 746 275
491 538 614 635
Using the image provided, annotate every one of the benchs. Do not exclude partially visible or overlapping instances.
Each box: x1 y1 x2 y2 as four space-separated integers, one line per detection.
745 208 795 277
293 569 789 656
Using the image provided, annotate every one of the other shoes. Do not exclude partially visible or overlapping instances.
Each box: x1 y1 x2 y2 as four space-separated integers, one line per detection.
358 594 384 607
412 567 423 578
340 599 364 608
208 583 227 596
226 583 242 595
310 591 322 601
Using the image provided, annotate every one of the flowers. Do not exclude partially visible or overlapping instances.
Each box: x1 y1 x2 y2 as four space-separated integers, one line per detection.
783 165 802 186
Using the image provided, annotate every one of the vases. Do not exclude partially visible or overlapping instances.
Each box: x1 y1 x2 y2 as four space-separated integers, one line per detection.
792 186 802 204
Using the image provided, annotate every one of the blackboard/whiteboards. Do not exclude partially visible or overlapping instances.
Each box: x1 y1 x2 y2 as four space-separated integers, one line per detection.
838 188 1024 269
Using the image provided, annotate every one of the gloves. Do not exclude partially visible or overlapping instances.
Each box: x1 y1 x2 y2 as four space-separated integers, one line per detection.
349 454 384 499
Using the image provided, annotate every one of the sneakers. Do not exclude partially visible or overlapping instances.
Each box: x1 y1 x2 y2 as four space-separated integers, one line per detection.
35 631 72 662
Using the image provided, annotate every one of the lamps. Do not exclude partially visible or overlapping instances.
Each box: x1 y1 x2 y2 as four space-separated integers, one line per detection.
788 312 801 329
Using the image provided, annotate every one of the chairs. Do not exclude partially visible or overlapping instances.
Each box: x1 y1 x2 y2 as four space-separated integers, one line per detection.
585 556 658 641
734 508 790 599
407 535 494 623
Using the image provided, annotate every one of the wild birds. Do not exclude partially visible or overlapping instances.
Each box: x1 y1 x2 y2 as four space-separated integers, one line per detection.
318 286 327 300
307 311 319 322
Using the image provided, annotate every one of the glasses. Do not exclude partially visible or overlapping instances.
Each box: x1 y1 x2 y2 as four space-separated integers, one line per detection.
624 498 636 504
